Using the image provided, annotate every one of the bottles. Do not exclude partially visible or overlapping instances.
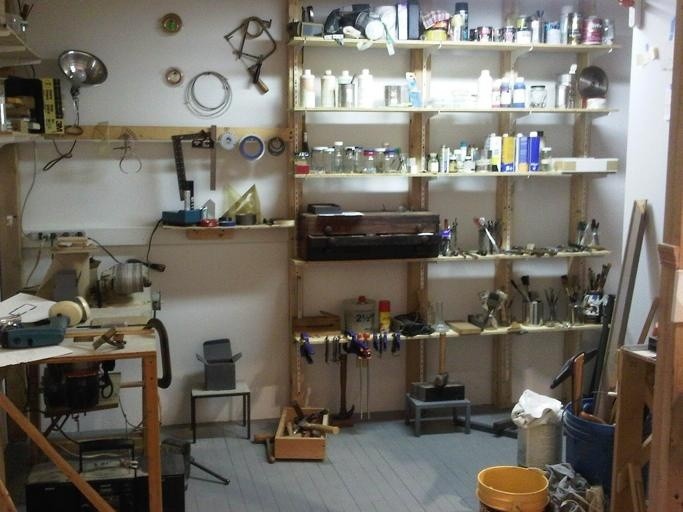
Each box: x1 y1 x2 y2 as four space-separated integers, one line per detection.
420 2 611 48
427 300 448 333
377 300 392 333
294 66 577 176
183 184 192 211
356 296 366 304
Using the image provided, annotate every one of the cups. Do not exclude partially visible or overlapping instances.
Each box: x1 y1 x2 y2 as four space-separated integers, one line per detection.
440 229 455 256
578 292 602 323
497 299 584 327
201 214 294 225
577 230 600 250
478 229 492 255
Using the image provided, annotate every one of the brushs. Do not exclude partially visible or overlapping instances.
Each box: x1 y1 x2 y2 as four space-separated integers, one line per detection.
512 276 539 302
478 288 509 329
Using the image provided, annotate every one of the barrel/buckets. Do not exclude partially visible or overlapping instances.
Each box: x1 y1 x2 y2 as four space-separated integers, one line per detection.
475 466 550 512
560 393 651 495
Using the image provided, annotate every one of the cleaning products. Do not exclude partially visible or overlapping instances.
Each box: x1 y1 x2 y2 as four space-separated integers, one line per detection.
476 69 494 104
300 69 376 108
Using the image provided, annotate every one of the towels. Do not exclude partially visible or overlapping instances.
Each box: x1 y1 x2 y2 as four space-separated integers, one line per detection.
509 386 565 429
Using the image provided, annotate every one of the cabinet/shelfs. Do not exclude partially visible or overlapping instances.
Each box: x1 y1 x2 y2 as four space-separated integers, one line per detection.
284 2 622 426
0 125 294 250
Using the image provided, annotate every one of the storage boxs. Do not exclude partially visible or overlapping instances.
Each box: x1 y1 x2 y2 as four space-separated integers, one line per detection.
272 405 330 463
26 441 189 511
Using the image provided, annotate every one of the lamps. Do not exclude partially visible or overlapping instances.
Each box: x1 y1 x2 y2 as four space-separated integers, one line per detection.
58 48 109 128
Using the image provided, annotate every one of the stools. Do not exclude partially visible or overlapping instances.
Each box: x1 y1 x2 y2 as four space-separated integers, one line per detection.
189 382 252 444
403 391 475 438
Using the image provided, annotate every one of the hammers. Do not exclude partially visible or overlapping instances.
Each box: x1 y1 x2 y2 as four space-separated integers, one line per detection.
293 415 340 436
255 434 275 464
433 332 449 388
332 342 354 421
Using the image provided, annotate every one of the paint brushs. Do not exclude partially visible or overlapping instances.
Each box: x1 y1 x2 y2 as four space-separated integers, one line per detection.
589 264 612 291
560 275 583 304
544 287 562 320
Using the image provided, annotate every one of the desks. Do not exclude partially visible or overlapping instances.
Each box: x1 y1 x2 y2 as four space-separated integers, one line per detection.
0 293 164 512
